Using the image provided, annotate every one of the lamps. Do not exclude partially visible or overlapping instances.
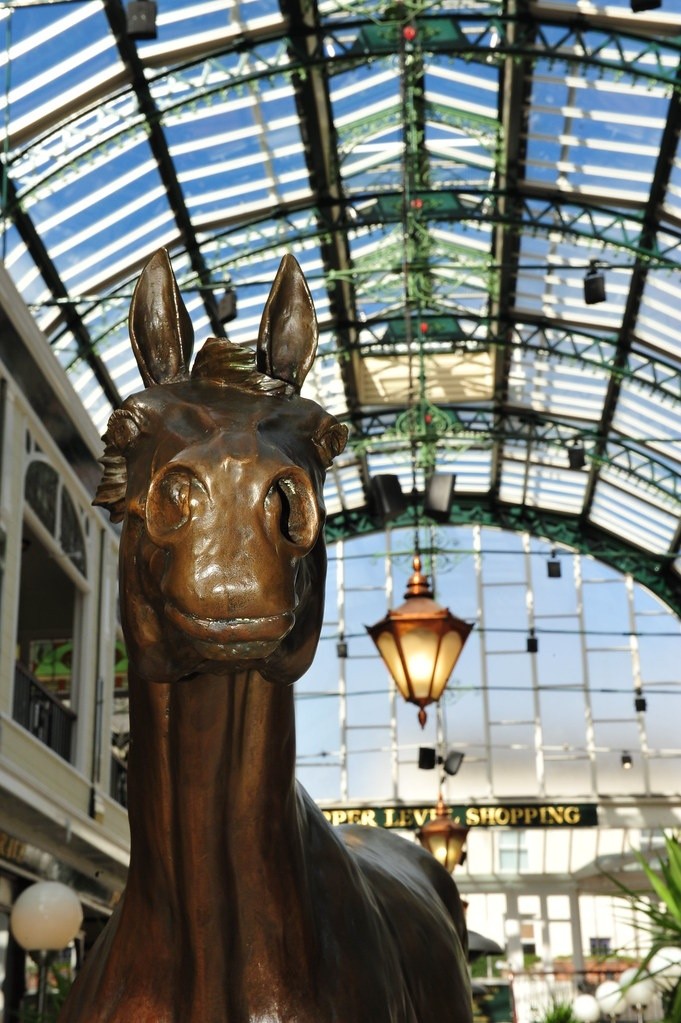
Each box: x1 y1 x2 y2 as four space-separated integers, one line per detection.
367 473 412 522
219 288 237 325
568 438 587 470
419 473 459 523
634 687 647 712
442 749 465 776
620 751 633 770
418 747 439 769
337 637 349 659
546 550 562 578
127 0 158 40
361 24 477 729
527 631 538 653
582 257 611 305
415 335 471 877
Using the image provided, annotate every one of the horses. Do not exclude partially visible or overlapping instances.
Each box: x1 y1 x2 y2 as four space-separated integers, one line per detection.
48 248 473 1023
